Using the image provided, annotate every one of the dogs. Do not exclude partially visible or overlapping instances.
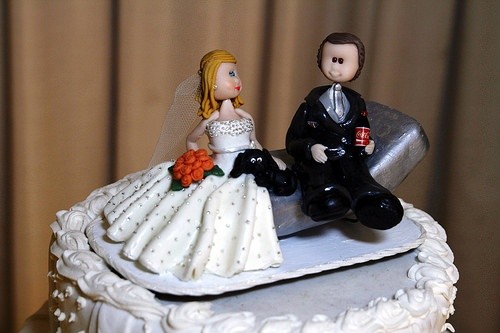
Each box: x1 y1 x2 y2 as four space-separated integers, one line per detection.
229 148 297 196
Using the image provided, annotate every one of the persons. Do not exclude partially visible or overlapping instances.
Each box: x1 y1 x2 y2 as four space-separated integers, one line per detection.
285 33 404 230
102 49 289 280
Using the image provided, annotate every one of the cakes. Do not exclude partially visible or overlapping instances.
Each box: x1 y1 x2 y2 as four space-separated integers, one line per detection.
19 168 459 333
